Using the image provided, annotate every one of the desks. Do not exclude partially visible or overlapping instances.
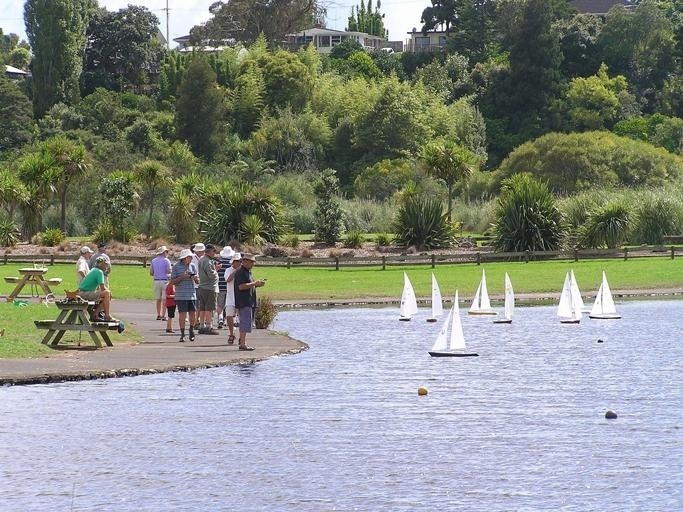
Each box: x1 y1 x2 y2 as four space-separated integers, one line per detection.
51 299 101 349
8 268 51 302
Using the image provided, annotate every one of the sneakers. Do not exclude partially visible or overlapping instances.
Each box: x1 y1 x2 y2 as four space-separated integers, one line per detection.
166 320 224 341
228 335 254 350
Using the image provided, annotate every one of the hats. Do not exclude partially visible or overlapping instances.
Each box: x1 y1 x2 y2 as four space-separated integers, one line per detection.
97 257 108 263
80 247 93 254
156 246 169 255
220 246 235 257
178 249 195 260
193 243 205 251
232 253 241 260
242 253 256 262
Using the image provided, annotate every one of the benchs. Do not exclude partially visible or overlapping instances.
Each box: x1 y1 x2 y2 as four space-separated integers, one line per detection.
88 320 119 347
2 275 18 303
42 276 62 299
32 318 57 350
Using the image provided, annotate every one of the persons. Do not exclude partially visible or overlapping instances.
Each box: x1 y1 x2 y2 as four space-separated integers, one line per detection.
196 244 220 336
233 252 265 351
189 242 206 273
214 244 236 330
77 256 117 322
163 273 175 334
148 245 171 322
75 245 89 287
188 244 195 254
222 253 243 345
87 242 111 291
169 248 200 343
